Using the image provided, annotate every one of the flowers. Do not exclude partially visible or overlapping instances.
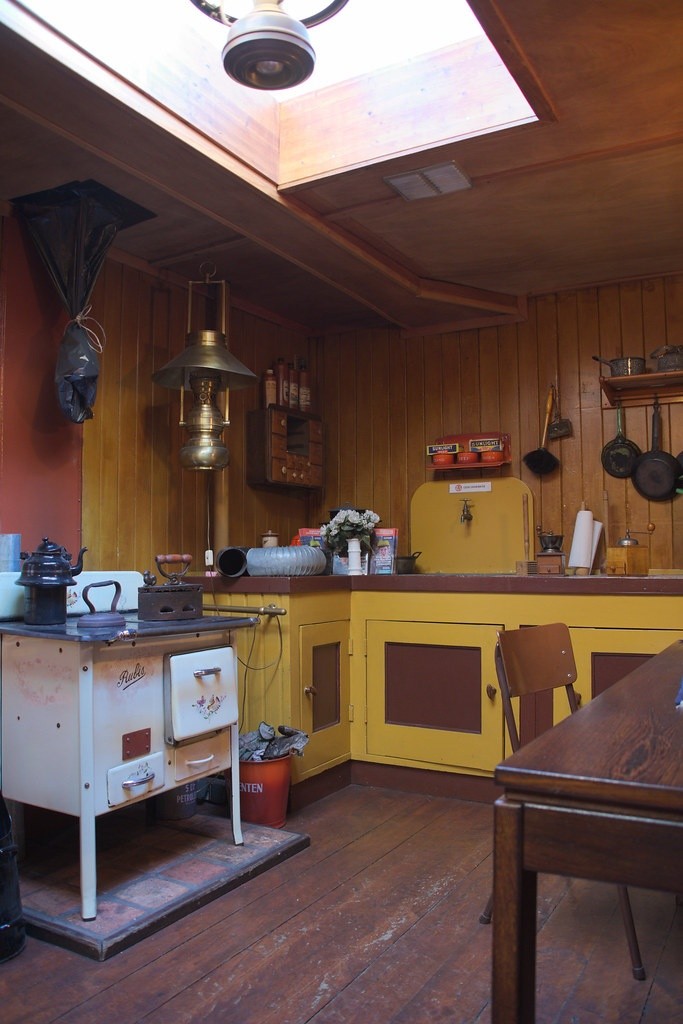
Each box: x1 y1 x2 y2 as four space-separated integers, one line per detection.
324 507 380 551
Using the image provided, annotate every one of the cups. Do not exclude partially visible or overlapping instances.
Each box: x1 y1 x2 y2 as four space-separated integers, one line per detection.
260 530 280 549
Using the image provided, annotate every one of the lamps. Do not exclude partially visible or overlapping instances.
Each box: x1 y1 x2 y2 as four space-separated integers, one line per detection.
149 268 259 472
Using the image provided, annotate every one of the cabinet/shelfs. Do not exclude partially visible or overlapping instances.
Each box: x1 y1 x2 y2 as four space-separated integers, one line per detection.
195 589 356 785
246 406 325 492
345 596 683 778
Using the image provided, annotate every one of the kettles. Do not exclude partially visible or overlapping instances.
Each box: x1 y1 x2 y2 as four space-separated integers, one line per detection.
651 345 683 373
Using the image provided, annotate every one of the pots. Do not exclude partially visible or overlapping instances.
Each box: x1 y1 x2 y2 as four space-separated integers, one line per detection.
601 401 641 478
395 550 422 574
592 355 644 376
631 401 683 501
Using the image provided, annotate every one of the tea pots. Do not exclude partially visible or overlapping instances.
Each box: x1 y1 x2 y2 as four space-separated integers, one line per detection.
13 536 88 625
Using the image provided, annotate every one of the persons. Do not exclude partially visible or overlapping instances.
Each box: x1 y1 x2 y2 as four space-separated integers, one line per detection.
372 540 392 569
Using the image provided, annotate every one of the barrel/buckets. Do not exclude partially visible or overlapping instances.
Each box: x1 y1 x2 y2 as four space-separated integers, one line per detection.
224 751 292 829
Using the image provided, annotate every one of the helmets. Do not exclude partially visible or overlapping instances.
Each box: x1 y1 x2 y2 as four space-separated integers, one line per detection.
377 540 389 547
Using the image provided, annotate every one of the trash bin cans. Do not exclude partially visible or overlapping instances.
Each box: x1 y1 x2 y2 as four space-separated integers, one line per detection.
240 748 297 828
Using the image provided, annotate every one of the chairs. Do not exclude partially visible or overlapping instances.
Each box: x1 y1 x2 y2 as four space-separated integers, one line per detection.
480 622 645 981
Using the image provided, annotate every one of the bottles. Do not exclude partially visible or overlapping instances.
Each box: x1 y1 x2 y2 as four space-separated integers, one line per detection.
262 370 277 408
300 363 312 412
273 357 290 409
286 362 299 410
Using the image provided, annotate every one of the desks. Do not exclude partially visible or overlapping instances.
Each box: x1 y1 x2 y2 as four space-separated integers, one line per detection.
480 637 683 1024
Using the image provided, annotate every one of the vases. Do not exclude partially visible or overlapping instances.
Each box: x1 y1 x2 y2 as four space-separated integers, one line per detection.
348 538 363 573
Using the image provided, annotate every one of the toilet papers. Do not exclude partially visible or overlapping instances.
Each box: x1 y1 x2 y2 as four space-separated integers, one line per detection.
567 510 603 575
